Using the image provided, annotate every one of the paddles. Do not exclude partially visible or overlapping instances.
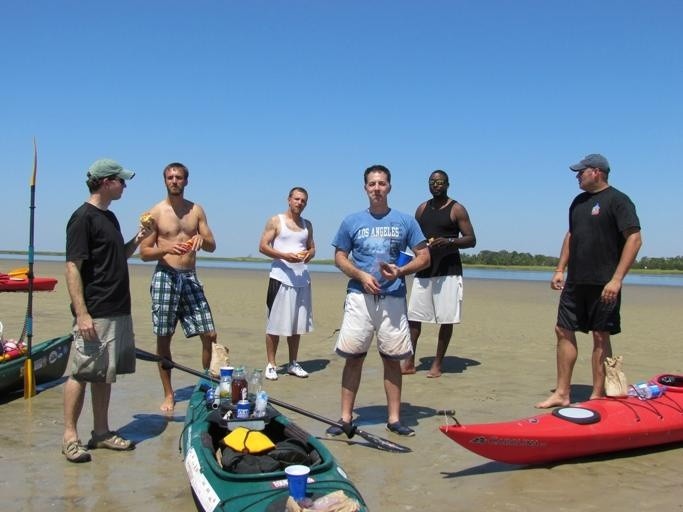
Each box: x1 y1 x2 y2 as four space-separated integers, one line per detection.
136 348 411 452
1 268 29 281
23 138 37 402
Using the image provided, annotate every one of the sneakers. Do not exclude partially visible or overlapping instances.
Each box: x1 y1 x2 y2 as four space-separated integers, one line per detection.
63 440 90 462
89 430 132 450
287 360 309 378
384 420 415 437
265 362 278 380
325 416 354 436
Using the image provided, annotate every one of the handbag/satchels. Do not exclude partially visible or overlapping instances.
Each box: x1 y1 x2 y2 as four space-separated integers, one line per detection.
604 355 629 398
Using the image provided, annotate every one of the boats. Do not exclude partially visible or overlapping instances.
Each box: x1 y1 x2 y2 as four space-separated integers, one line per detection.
0 332 74 399
0 273 58 292
182 368 369 512
440 371 683 465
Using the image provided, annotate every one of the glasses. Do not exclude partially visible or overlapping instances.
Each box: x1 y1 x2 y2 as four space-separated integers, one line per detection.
429 178 445 185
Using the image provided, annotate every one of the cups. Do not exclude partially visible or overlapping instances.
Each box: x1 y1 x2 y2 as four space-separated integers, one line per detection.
284 463 311 502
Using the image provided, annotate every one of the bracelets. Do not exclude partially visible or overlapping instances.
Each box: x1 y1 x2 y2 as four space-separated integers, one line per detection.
446 237 455 249
554 269 564 273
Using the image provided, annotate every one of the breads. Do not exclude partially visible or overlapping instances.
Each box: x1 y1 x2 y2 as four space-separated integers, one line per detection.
296 252 306 262
182 240 193 250
140 213 153 226
283 489 360 512
429 237 434 244
379 261 394 275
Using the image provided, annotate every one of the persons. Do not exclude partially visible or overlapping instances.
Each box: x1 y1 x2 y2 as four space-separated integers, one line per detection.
534 154 642 410
399 170 477 377
324 164 433 437
260 187 316 380
140 162 217 411
60 159 158 463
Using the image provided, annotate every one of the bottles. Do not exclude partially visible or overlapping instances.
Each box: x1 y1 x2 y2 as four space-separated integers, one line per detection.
626 378 665 402
205 362 270 422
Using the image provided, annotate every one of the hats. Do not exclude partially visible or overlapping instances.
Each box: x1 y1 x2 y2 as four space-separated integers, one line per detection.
85 159 136 182
570 154 610 175
